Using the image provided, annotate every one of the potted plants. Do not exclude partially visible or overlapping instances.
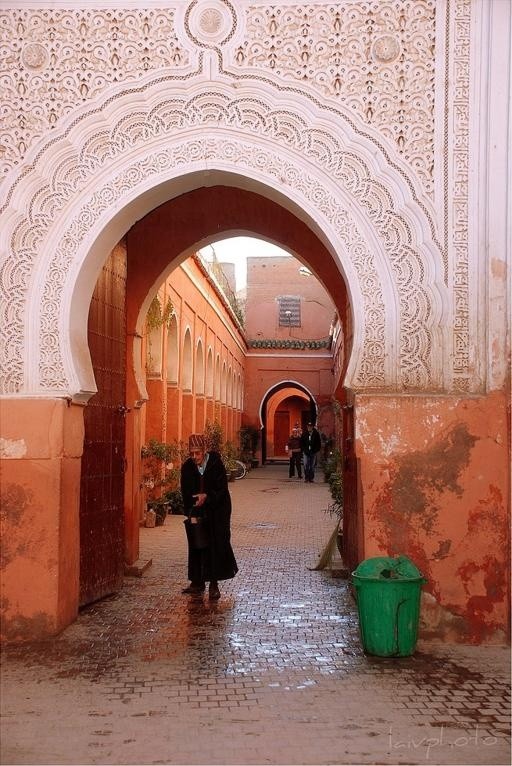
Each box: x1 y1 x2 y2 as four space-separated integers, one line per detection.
140 440 186 528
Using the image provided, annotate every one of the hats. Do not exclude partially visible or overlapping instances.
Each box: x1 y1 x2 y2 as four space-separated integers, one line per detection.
307 422 313 425
188 435 204 452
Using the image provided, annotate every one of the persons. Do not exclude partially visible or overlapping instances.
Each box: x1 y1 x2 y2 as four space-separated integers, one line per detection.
181 434 239 600
300 421 321 483
284 428 303 479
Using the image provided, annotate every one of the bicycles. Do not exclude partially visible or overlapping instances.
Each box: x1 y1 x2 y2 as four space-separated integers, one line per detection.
219 449 247 480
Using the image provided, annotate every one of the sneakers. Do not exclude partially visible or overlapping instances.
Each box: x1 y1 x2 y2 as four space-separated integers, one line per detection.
289 475 314 483
182 581 205 592
209 583 220 598
